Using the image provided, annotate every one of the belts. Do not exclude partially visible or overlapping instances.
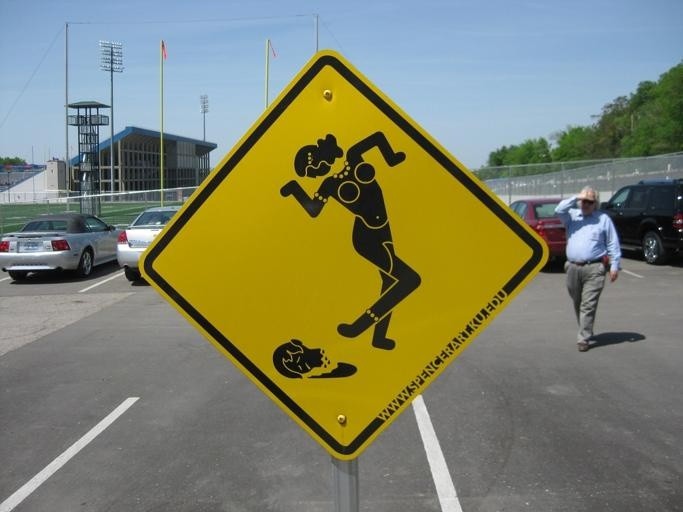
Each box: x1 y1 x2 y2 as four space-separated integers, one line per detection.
573 259 602 266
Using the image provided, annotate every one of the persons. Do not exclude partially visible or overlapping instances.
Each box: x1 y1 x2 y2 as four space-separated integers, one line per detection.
554 188 622 350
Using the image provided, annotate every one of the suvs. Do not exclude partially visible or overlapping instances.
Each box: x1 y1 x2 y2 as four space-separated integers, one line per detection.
598 178 683 265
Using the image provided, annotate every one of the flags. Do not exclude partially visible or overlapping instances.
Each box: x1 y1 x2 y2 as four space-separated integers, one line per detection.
270 42 277 57
162 45 168 60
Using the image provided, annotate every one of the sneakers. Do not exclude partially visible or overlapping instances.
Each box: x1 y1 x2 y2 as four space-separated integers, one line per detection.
577 342 588 351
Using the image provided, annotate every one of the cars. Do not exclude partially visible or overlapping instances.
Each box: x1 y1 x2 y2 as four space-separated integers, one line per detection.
508 198 579 265
0 205 183 281
